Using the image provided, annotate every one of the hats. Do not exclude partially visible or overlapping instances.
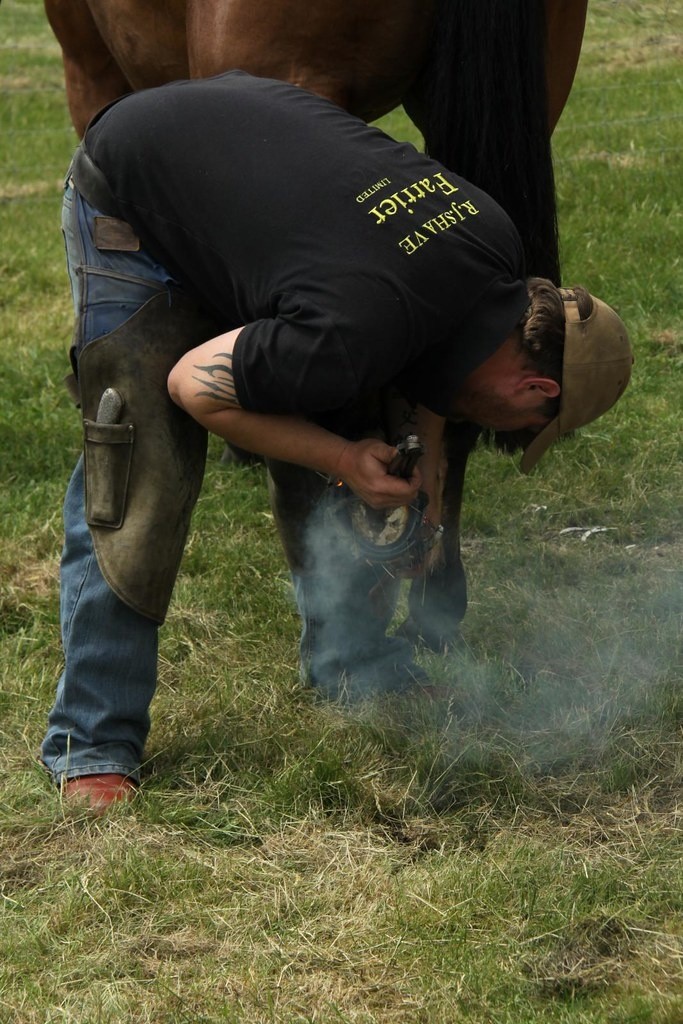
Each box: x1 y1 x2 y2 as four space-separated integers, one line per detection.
519 286 634 474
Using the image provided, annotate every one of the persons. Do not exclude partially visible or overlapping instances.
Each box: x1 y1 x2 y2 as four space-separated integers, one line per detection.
41 70 635 810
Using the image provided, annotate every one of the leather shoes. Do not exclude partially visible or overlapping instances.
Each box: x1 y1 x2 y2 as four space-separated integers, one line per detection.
64 774 135 812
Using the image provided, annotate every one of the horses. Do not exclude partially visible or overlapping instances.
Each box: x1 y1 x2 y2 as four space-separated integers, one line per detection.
35 2 592 655
